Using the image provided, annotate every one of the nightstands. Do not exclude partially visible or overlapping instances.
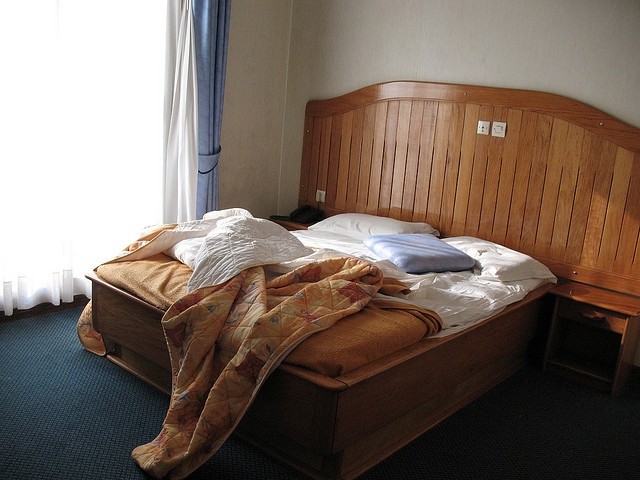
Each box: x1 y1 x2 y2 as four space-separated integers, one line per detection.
535 279 639 403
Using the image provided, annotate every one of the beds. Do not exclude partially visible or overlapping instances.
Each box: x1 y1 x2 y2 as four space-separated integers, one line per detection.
76 80 639 480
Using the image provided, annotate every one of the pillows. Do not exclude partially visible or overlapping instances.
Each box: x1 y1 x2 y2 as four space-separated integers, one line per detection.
439 235 558 284
361 232 476 276
306 213 440 244
